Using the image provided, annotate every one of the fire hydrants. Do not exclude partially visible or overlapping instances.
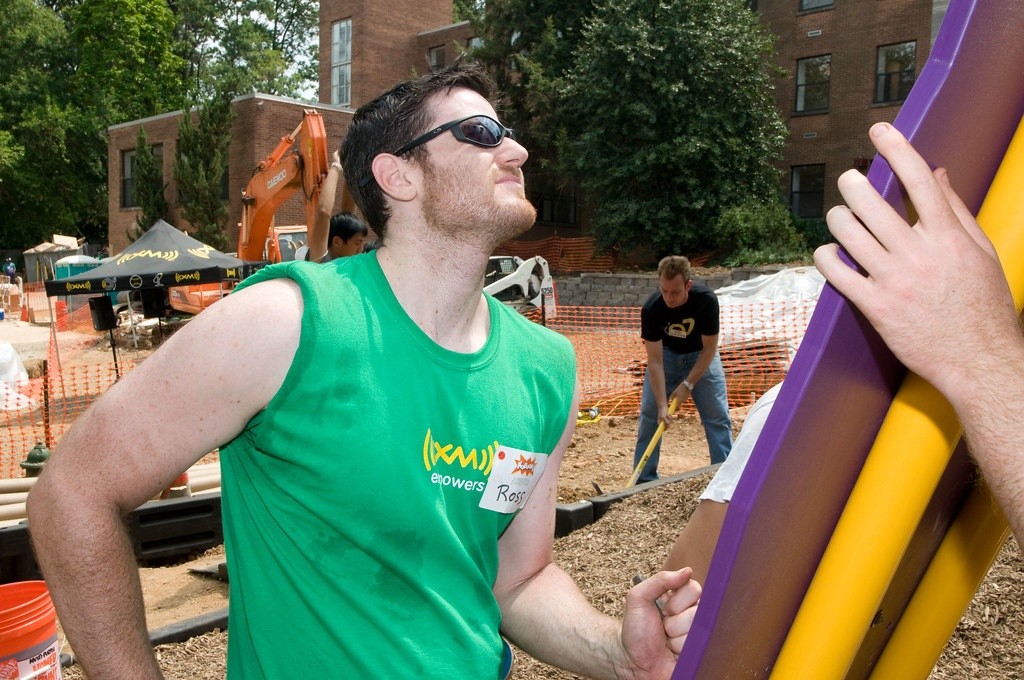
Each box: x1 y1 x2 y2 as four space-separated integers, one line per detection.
18 442 53 477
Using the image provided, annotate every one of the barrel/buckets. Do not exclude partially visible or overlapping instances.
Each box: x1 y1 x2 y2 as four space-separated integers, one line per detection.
0 581 63 680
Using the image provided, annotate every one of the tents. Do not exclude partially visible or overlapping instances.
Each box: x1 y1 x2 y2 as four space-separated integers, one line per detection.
44 218 272 399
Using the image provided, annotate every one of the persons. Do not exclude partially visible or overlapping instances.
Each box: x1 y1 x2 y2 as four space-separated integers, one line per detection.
634 255 733 485
322 211 368 264
1 257 16 285
813 122 1024 555
25 52 703 680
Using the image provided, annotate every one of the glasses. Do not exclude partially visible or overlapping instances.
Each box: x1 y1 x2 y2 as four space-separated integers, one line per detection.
359 114 518 188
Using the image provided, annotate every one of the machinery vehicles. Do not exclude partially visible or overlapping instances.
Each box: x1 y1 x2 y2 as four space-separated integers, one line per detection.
165 108 329 314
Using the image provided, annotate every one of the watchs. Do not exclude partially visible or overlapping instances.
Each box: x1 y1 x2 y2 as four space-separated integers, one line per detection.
682 378 694 391
